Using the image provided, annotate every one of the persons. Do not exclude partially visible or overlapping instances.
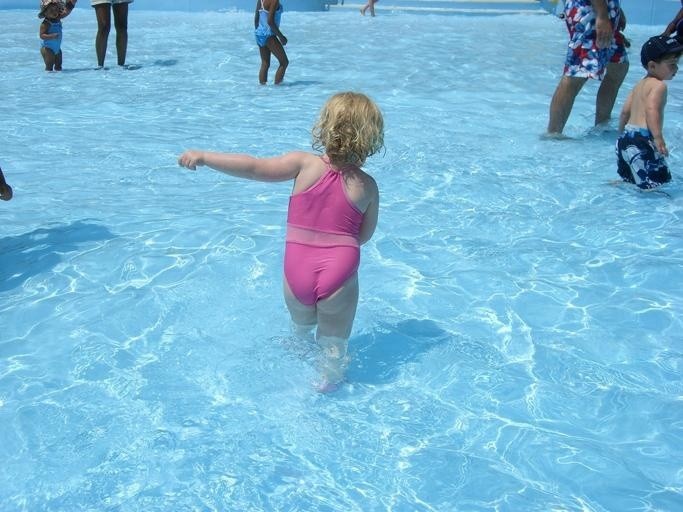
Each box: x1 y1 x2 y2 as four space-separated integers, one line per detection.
252 0 290 85
175 90 389 394
88 0 134 70
36 1 77 73
358 0 378 17
660 0 682 57
0 166 13 202
545 0 631 136
614 35 680 192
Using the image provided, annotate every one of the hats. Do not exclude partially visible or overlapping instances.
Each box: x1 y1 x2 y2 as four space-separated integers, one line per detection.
37 0 66 18
640 36 683 66
